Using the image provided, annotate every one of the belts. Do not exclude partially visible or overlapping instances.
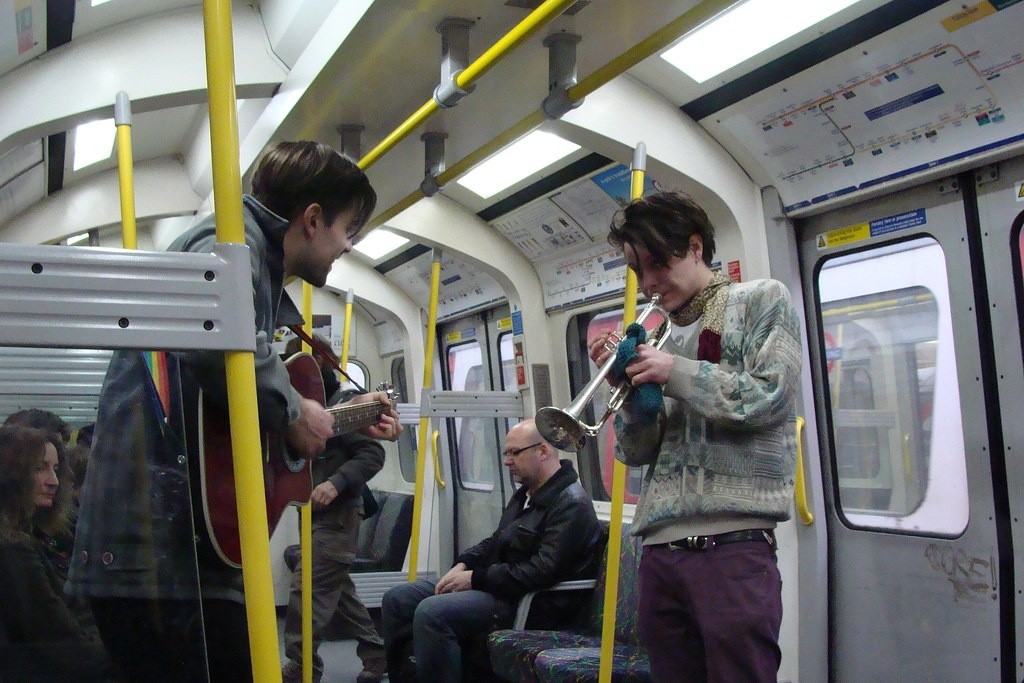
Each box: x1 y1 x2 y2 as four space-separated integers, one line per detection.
654 528 774 550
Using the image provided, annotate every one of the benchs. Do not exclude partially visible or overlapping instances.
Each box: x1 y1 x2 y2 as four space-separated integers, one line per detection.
487 516 650 683
284 489 415 573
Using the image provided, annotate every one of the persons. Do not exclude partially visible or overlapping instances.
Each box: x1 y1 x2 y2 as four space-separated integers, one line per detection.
380 414 603 683
586 175 803 683
1 137 405 683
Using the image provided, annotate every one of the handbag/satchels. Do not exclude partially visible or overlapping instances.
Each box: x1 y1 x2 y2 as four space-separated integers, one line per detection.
358 487 380 518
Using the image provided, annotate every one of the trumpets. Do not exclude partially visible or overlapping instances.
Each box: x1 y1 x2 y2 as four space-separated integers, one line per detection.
537 294 675 451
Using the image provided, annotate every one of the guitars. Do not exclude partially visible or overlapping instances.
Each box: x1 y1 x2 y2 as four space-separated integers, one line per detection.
187 352 396 572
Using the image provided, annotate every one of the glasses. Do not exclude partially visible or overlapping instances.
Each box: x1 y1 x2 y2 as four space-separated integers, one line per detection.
504 441 541 456
629 244 692 279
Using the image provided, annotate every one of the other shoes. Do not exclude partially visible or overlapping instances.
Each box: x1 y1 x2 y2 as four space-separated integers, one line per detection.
283 661 323 683
357 658 388 683
